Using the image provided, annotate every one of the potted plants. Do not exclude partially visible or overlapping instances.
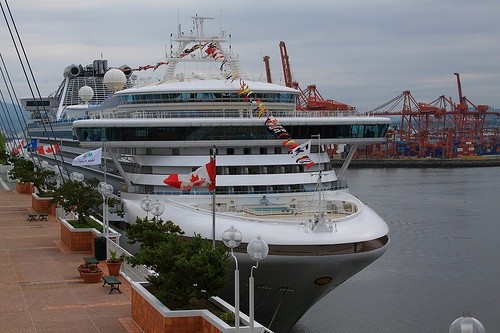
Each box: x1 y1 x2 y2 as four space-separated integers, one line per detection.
105 249 123 275
48 177 125 256
20 168 57 216
124 216 264 333
9 157 38 194
78 264 103 282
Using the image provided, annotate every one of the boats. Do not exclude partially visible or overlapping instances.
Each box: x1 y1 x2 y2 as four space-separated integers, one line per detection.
5 7 391 333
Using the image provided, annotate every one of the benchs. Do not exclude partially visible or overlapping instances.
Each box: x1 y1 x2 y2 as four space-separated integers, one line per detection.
83 257 99 269
37 212 48 221
28 213 37 221
102 275 122 294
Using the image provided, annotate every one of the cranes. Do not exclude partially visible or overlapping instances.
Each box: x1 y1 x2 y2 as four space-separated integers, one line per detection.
263 41 500 160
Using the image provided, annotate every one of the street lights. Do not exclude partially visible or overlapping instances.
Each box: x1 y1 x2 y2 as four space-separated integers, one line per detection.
222 226 269 333
23 154 165 238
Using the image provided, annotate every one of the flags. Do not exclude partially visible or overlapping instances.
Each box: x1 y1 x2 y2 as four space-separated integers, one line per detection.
164 160 216 190
17 140 24 149
133 42 314 169
72 148 101 166
39 144 58 154
23 140 38 148
